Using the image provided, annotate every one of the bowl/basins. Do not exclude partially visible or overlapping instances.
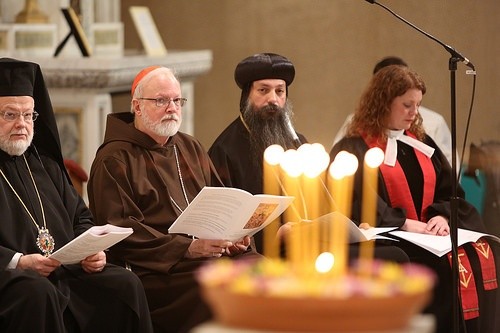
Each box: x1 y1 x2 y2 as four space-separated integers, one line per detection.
194 258 436 333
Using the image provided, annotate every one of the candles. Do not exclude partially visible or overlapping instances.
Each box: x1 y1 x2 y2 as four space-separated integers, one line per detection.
262 141 387 291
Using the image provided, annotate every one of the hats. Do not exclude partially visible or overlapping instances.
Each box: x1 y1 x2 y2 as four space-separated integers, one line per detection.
0 57 65 167
234 53 295 89
131 66 161 95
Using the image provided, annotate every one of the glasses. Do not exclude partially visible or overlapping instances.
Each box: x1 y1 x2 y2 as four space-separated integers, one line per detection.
0 109 39 122
136 96 188 109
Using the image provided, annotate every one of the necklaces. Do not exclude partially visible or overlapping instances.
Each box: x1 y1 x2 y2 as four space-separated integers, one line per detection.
169 144 197 241
0 153 54 257
241 114 310 222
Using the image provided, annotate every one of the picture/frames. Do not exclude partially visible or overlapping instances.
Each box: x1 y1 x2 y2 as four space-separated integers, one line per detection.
127 5 168 58
52 6 93 59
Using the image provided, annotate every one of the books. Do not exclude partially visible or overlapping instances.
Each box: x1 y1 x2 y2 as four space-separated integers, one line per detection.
168 186 294 243
388 228 500 257
49 223 134 265
311 212 400 244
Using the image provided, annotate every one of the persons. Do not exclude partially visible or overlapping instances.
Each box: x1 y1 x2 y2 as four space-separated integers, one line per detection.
334 56 460 186
0 56 153 333
87 66 268 333
329 65 500 333
208 52 409 263
